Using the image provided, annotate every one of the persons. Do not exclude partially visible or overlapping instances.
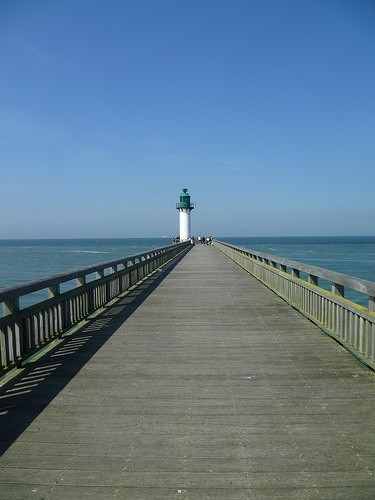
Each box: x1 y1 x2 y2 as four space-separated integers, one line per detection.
173 234 213 247
